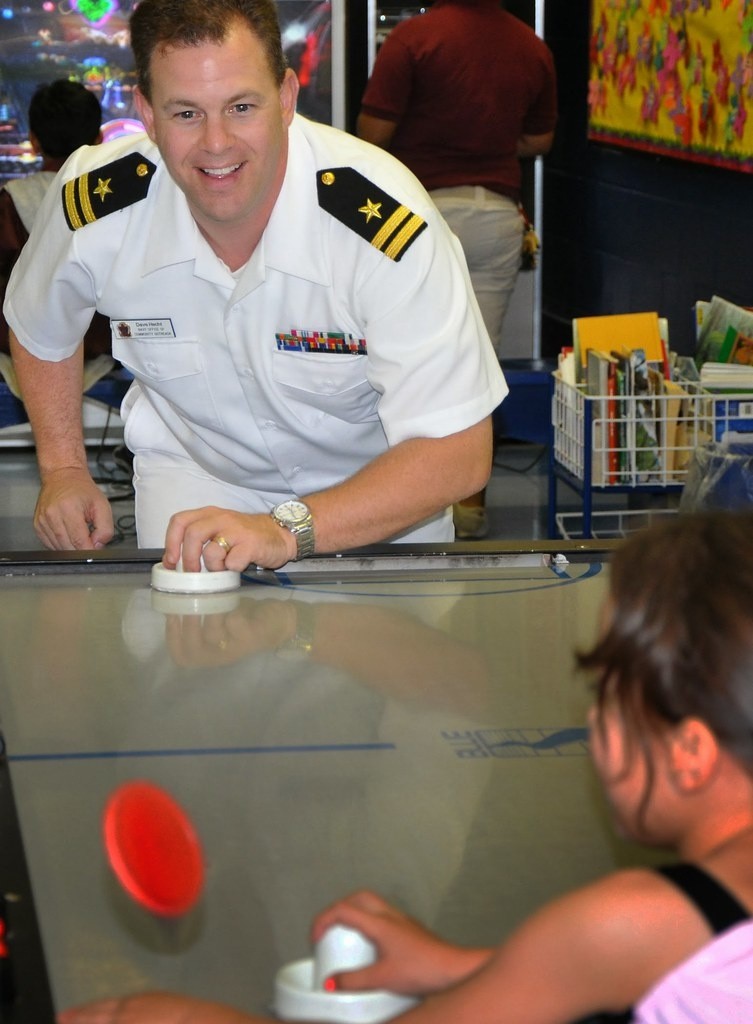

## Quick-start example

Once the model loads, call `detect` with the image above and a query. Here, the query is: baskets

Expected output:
[550,370,753,486]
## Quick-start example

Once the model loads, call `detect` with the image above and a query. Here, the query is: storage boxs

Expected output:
[493,358,556,441]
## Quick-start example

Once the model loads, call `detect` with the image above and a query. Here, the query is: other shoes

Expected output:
[453,502,489,539]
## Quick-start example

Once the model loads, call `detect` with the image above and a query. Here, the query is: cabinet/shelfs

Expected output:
[547,369,753,539]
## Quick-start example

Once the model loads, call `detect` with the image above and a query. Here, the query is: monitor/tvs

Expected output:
[0,0,345,183]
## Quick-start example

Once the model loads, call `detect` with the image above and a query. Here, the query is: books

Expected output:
[559,295,753,485]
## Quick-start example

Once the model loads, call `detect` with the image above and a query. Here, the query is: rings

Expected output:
[211,536,231,553]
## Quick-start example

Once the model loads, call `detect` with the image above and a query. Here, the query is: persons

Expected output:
[0,0,557,572]
[58,506,753,1024]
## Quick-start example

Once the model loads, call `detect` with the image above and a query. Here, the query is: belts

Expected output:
[486,182,521,202]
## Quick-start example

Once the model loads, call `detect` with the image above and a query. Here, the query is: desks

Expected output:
[0,539,753,1024]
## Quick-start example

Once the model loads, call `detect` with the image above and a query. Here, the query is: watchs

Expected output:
[270,498,316,562]
